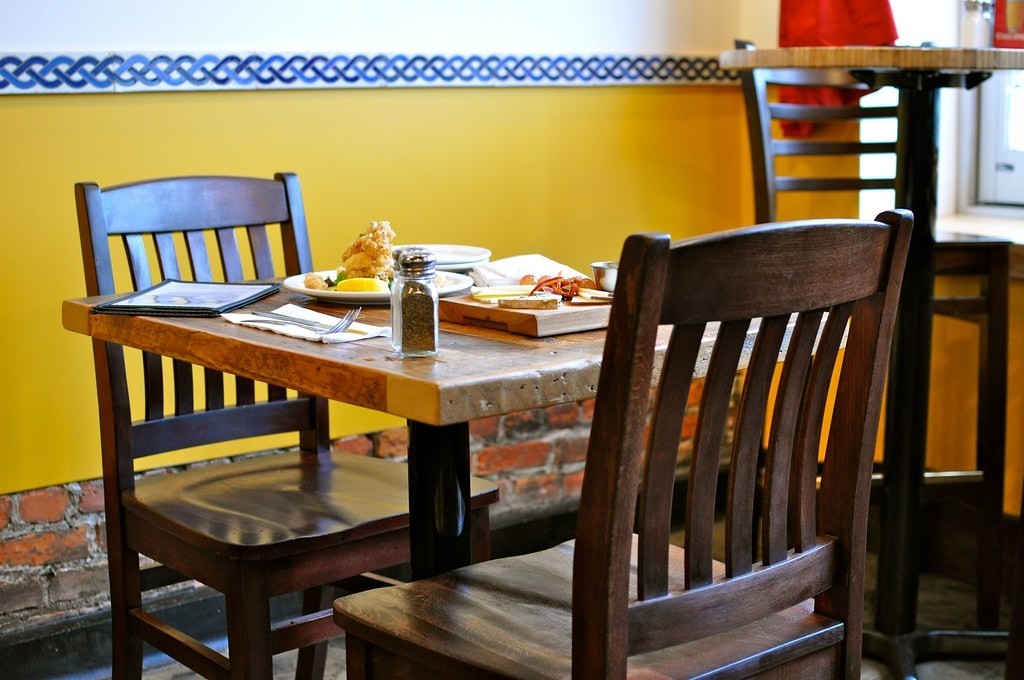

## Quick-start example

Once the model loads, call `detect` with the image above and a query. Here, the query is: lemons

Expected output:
[337,278,388,292]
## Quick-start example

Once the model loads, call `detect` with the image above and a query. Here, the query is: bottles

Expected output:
[960,0,995,48]
[390,247,440,358]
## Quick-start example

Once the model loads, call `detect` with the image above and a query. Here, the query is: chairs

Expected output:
[74,171,504,680]
[733,38,1016,665]
[329,207,919,680]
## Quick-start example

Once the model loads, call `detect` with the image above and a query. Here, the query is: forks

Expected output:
[240,306,363,335]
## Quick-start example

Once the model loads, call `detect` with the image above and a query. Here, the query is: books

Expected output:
[89,278,281,317]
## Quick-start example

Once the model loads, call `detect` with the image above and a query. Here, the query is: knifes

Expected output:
[251,311,368,336]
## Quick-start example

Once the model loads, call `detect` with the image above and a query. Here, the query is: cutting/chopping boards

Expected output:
[435,288,613,338]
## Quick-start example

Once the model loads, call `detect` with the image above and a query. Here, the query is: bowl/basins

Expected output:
[589,261,620,292]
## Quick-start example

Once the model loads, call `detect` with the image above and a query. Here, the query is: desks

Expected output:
[62,274,850,576]
[720,43,1024,680]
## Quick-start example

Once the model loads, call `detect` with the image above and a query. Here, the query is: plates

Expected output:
[392,245,492,272]
[282,270,475,305]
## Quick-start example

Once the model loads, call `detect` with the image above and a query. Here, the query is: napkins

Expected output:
[470,253,589,288]
[219,301,392,345]
